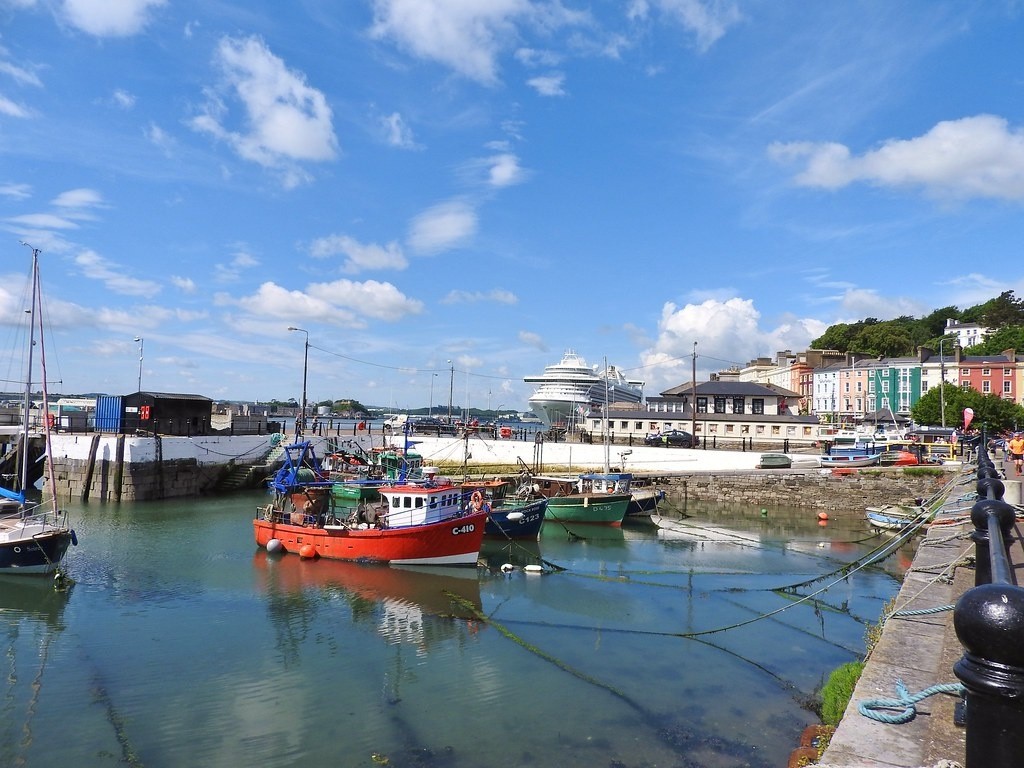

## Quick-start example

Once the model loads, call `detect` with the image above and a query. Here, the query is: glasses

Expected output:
[1015,436,1020,437]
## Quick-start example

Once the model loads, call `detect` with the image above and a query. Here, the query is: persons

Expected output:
[987,433,1024,475]
[294,413,318,436]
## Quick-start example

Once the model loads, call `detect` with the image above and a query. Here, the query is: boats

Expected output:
[253,356,666,569]
[819,441,945,468]
[863,506,932,531]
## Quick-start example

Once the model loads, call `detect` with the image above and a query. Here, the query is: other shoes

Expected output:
[1019,468,1022,472]
[1017,472,1019,476]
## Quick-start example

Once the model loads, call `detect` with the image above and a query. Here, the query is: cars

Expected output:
[643,430,700,449]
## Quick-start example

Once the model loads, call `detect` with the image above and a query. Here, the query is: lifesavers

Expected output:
[485,502,492,513]
[470,491,483,509]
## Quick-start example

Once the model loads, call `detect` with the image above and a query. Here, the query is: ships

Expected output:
[522,348,647,432]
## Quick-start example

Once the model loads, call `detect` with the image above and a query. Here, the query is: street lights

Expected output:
[447,359,454,424]
[287,326,308,435]
[428,373,439,418]
[23,309,33,432]
[939,337,956,428]
[851,356,856,442]
[691,341,698,449]
[134,337,143,393]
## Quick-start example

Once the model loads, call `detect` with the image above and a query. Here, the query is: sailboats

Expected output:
[0,239,77,578]
